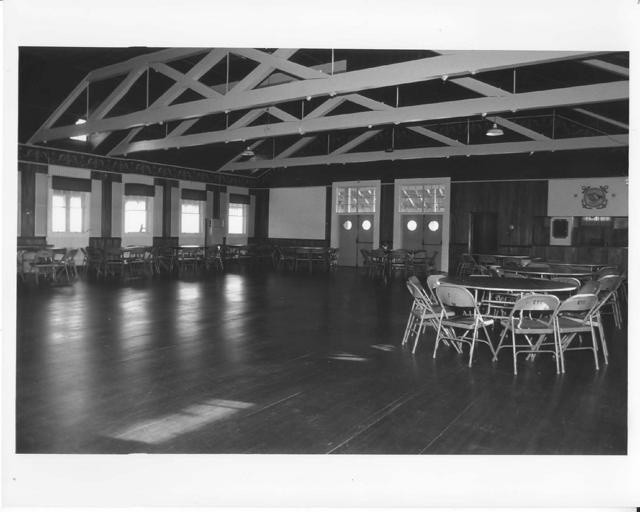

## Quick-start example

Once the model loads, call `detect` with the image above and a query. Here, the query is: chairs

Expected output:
[434,285,498,369]
[409,273,448,337]
[401,281,461,358]
[457,252,625,329]
[16,246,342,289]
[492,294,561,373]
[358,247,439,277]
[532,291,599,373]
[574,279,611,366]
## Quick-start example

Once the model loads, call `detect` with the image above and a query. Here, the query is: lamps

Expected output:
[240,146,255,156]
[485,117,504,137]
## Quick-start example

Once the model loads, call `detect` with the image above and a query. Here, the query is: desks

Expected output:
[465,277,577,345]
